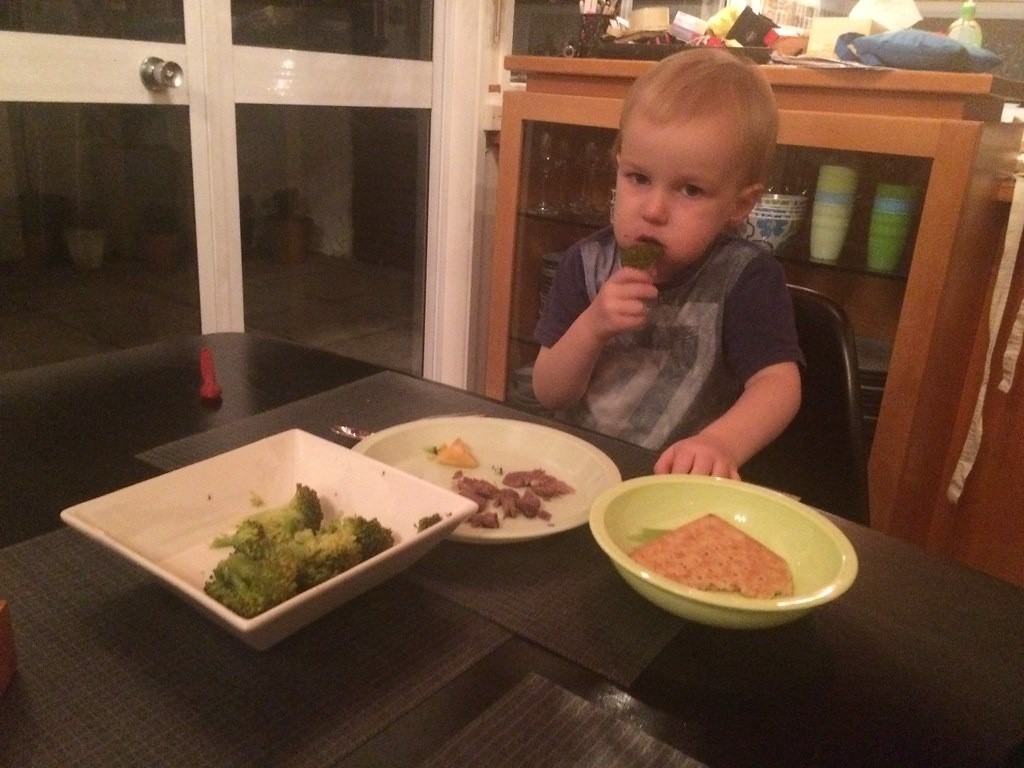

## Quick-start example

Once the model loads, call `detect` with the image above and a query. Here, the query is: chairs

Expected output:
[746,285,873,528]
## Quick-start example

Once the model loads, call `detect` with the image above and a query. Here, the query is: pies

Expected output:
[629,513,794,602]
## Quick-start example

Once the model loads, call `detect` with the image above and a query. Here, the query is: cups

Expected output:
[580,13,614,59]
[868,183,921,273]
[809,163,857,260]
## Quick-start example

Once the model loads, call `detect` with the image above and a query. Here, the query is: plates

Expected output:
[351,417,622,543]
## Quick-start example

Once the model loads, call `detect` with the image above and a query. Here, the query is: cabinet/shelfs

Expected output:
[488,54,1024,588]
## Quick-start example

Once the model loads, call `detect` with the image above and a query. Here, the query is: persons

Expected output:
[531,47,803,479]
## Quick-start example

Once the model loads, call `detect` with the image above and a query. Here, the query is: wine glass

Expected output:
[528,135,614,218]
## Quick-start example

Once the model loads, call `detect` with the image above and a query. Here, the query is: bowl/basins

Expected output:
[590,474,858,626]
[739,193,809,256]
[58,429,477,650]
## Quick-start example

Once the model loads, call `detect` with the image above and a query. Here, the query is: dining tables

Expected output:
[0,333,1023,768]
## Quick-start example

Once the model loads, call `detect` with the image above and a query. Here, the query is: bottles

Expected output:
[946,0,983,50]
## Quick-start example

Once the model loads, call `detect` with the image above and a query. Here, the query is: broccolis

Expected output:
[619,242,662,273]
[205,484,444,618]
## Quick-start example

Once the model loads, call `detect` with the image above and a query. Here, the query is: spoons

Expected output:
[328,424,370,441]
